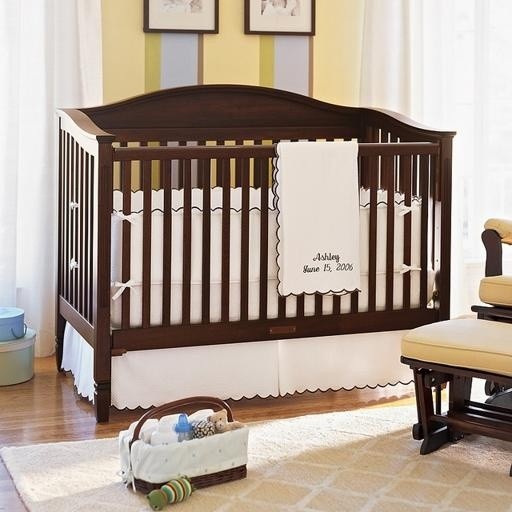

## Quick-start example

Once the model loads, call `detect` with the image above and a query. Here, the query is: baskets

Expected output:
[119,395,248,496]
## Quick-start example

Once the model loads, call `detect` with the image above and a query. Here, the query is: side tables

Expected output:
[400,318,512,477]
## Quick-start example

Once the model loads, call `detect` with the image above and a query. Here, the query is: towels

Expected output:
[271,141,362,298]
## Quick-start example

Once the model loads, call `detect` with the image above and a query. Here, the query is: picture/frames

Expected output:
[143,0,219,34]
[244,0,316,36]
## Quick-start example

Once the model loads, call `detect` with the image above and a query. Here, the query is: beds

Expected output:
[53,84,456,423]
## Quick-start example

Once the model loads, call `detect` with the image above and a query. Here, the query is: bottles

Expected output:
[176,415,192,442]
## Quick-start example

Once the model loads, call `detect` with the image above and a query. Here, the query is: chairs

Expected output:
[471,218,512,403]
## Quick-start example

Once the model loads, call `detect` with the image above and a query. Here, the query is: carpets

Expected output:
[0,403,512,512]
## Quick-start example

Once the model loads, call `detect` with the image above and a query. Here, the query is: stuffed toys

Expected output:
[145,475,196,510]
[206,409,243,433]
